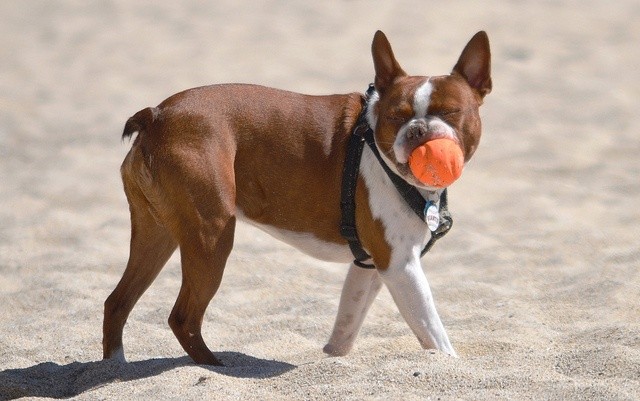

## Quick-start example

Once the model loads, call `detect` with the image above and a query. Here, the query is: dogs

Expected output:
[102,31,492,366]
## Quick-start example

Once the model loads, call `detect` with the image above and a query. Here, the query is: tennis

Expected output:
[409,138,465,187]
